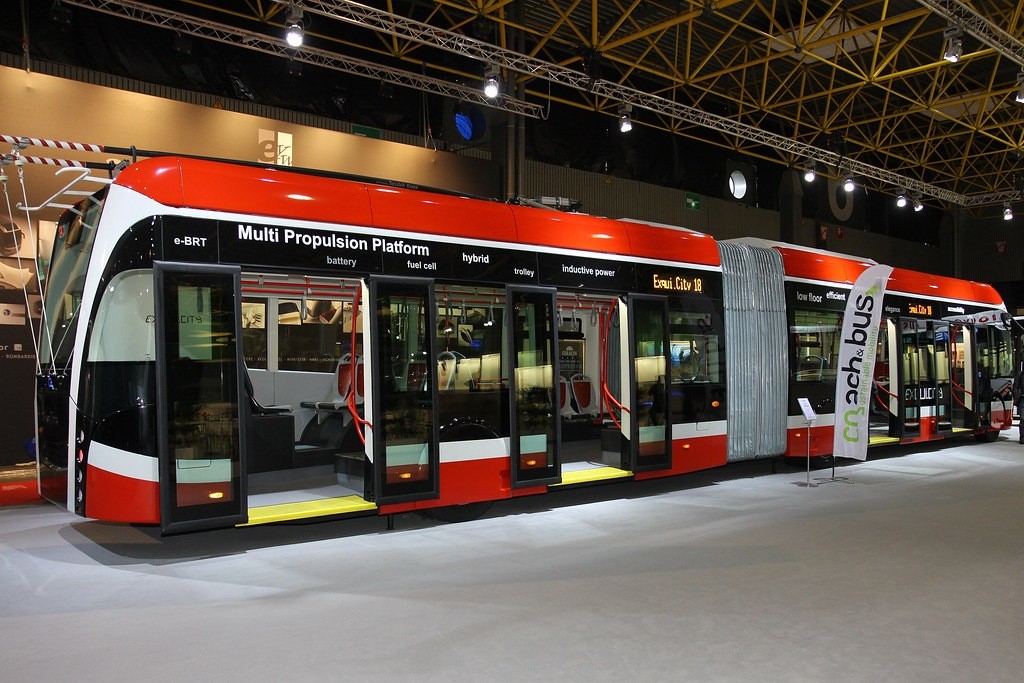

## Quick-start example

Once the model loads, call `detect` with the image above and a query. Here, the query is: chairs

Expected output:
[560,375,579,419]
[300,353,357,424]
[402,351,427,391]
[317,354,364,427]
[569,373,613,417]
[424,351,457,391]
[797,355,824,381]
[688,374,713,384]
[819,355,831,379]
[244,361,294,416]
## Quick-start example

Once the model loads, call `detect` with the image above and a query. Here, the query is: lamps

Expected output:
[913,199,923,212]
[844,179,855,192]
[896,195,906,208]
[286,25,305,48]
[1003,200,1013,220]
[804,169,815,183]
[619,117,633,133]
[484,78,498,98]
[1016,72,1024,103]
[942,18,963,62]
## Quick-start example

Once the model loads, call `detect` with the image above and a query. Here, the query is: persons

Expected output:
[648,377,665,425]
[1013,370,1024,444]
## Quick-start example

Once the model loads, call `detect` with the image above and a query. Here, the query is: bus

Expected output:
[0,133,1024,540]
